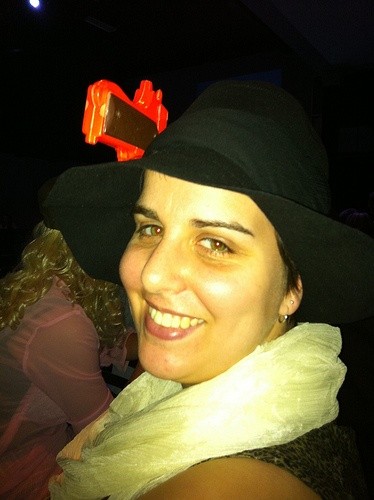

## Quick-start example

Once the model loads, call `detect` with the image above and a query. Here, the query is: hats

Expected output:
[36,78,373,325]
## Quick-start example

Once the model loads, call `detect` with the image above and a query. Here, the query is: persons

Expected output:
[38,76,374,499]
[1,218,144,499]
